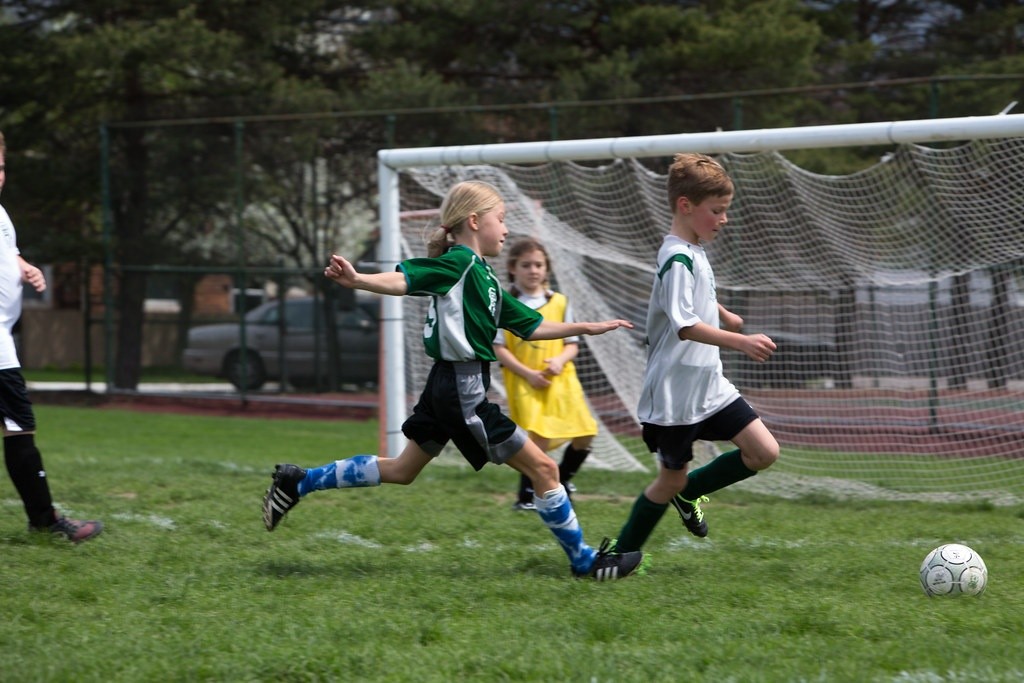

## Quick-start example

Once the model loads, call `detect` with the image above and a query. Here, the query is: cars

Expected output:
[182,287,379,396]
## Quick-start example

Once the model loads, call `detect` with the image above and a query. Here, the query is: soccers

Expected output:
[919,543,988,601]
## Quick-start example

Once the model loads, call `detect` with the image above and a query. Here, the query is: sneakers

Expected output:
[572,538,642,582]
[28,511,100,543]
[670,492,710,538]
[262,464,308,531]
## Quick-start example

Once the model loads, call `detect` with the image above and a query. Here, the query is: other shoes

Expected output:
[516,501,536,511]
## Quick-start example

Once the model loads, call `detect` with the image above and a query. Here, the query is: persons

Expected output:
[601,155,780,555]
[492,237,599,510]
[0,131,102,544]
[262,182,645,581]
